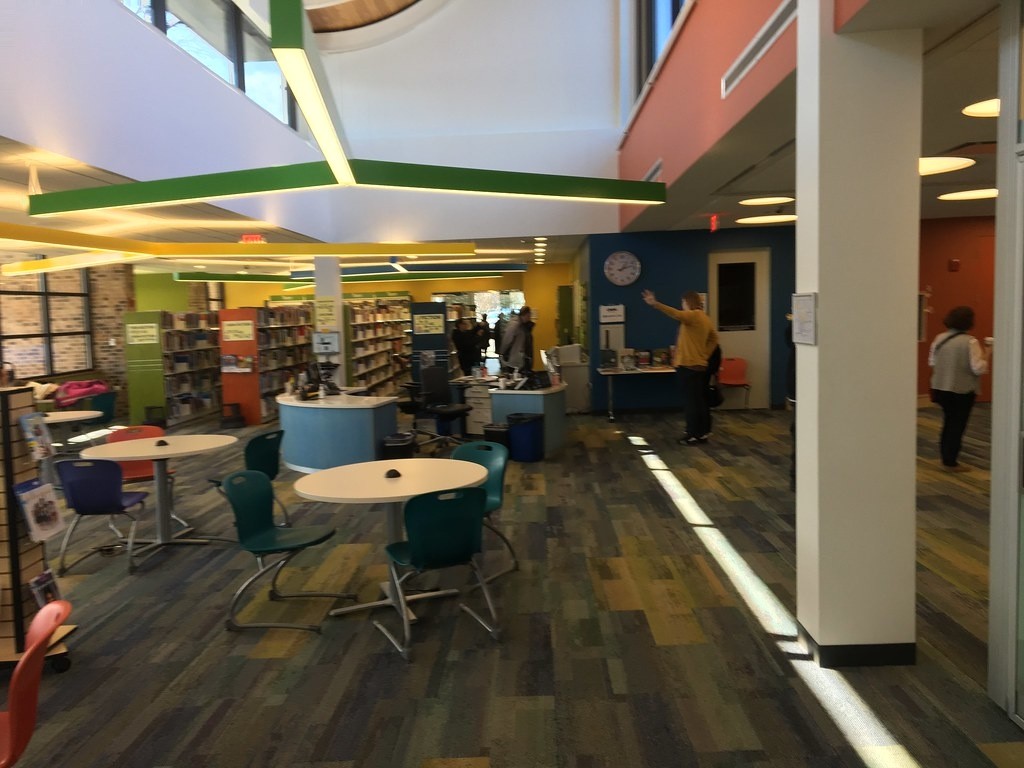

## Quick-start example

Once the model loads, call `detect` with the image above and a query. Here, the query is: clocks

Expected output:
[604,250,641,285]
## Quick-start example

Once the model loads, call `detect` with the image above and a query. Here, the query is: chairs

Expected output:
[0,382,518,768]
[714,357,752,413]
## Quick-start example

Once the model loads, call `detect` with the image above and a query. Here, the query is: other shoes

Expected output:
[945,463,971,473]
[680,432,708,445]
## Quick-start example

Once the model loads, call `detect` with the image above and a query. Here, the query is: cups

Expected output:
[498,378,507,389]
[983,337,992,347]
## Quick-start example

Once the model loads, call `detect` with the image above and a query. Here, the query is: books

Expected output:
[162,312,221,351]
[353,336,412,388]
[18,412,57,462]
[164,349,222,418]
[446,305,477,319]
[14,477,66,540]
[255,305,314,348]
[616,345,677,367]
[350,305,412,340]
[258,346,314,417]
[29,569,65,608]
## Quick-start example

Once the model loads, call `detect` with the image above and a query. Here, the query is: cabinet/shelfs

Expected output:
[448,376,568,460]
[124,296,476,427]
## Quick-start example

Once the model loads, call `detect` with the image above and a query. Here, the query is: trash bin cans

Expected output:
[385,432,413,459]
[507,412,544,462]
[435,384,466,435]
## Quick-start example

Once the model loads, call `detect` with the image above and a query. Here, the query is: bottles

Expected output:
[318,384,326,398]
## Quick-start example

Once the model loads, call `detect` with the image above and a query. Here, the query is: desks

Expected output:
[42,411,103,453]
[596,365,678,422]
[275,387,399,474]
[293,457,507,664]
[79,434,239,574]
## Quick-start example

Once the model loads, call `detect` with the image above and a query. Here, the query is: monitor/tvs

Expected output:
[484,357,501,375]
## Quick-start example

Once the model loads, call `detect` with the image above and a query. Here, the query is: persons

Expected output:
[479,314,490,355]
[926,306,990,472]
[446,321,464,379]
[641,289,719,447]
[499,305,536,372]
[452,318,485,376]
[784,314,796,492]
[494,310,518,369]
